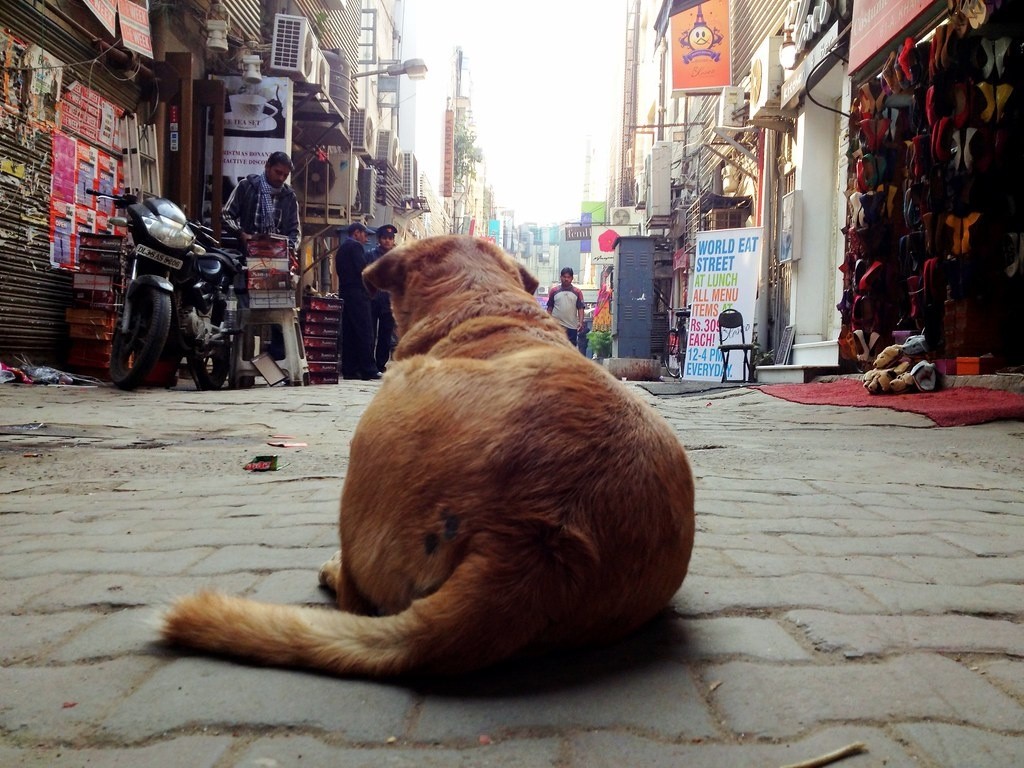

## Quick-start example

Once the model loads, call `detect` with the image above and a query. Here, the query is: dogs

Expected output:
[145,234,695,677]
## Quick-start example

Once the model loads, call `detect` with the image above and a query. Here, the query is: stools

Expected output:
[228,308,310,390]
[649,311,670,367]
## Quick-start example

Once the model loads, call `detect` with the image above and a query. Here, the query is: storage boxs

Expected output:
[301,295,344,384]
[956,356,1003,375]
[64,232,134,369]
[246,239,297,308]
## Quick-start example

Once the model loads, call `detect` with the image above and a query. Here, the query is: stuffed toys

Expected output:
[861,327,936,394]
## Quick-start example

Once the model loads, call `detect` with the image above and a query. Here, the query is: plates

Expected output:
[221,113,277,131]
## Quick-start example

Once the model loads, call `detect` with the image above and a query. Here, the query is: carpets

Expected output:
[635,379,770,401]
[748,377,1024,426]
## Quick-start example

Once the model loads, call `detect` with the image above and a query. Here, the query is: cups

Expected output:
[229,95,279,127]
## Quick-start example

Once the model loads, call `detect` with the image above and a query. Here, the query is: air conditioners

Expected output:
[609,205,645,236]
[403,151,420,209]
[269,12,318,83]
[376,130,403,179]
[749,36,785,117]
[712,86,744,142]
[291,155,359,203]
[293,48,331,111]
[349,109,378,157]
[645,140,672,222]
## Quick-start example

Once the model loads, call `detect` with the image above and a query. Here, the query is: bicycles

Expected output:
[661,310,692,378]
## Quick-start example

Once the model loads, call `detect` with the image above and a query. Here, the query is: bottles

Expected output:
[227,285,237,327]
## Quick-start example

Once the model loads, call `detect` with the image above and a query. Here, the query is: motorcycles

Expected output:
[83,185,248,391]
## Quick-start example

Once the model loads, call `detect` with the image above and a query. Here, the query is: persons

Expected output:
[363,223,397,372]
[335,223,386,379]
[576,319,590,356]
[546,267,585,347]
[220,151,303,389]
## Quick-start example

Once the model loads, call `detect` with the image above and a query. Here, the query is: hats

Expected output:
[376,224,397,236]
[349,222,376,236]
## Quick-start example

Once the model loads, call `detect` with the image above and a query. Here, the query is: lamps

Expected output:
[241,54,265,84]
[203,4,231,53]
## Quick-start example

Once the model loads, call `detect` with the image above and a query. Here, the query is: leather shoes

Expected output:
[371,372,385,379]
[343,375,361,380]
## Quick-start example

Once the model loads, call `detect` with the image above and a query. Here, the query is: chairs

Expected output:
[717,308,758,383]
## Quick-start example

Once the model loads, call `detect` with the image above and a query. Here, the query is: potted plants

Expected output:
[585,330,614,365]
